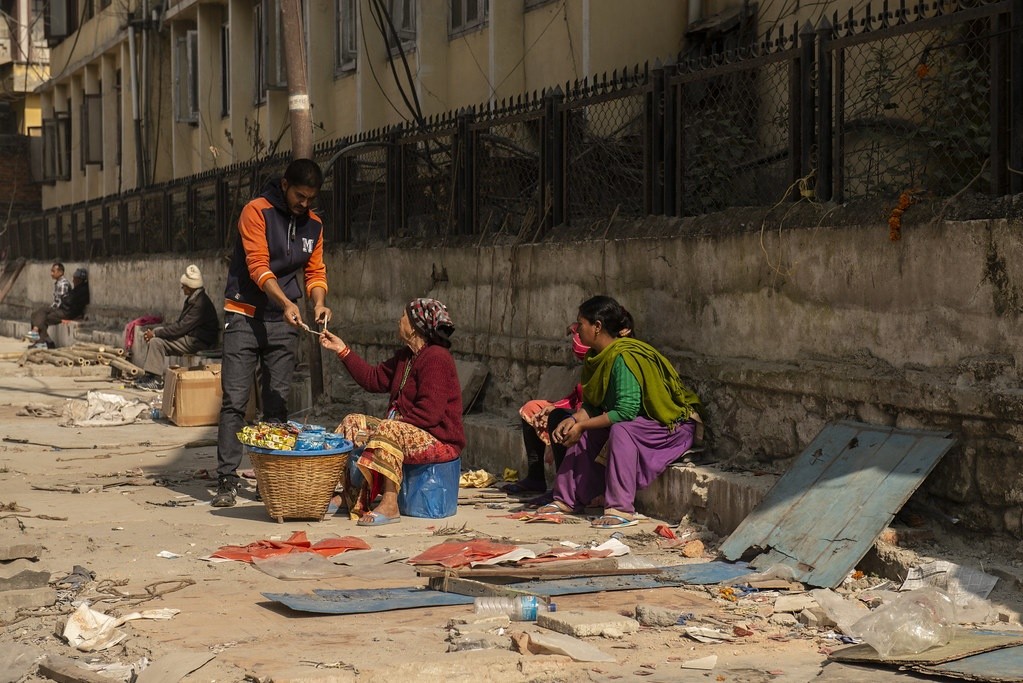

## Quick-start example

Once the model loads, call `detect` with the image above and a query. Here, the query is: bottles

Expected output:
[473,595,557,621]
[136,408,166,419]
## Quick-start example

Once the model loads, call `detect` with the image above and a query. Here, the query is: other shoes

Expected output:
[24,331,40,339]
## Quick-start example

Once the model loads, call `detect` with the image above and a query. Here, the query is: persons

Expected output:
[211,158,333,507]
[131,264,219,391]
[501,321,592,509]
[535,295,704,528]
[24,262,90,350]
[318,298,467,526]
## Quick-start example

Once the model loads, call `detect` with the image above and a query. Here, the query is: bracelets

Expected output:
[336,343,350,358]
[562,415,577,424]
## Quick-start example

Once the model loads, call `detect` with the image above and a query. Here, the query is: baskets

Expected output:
[245,439,353,523]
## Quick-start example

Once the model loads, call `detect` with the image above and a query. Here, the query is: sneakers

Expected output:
[136,378,164,392]
[255,484,263,500]
[211,476,238,506]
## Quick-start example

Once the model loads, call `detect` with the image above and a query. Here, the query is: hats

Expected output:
[73,269,86,280]
[181,264,203,288]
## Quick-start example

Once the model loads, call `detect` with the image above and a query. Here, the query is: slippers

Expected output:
[529,496,552,505]
[357,511,401,526]
[500,484,547,494]
[536,504,573,515]
[326,501,348,514]
[592,516,639,528]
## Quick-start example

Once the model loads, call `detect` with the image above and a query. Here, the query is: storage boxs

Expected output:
[161,364,263,427]
